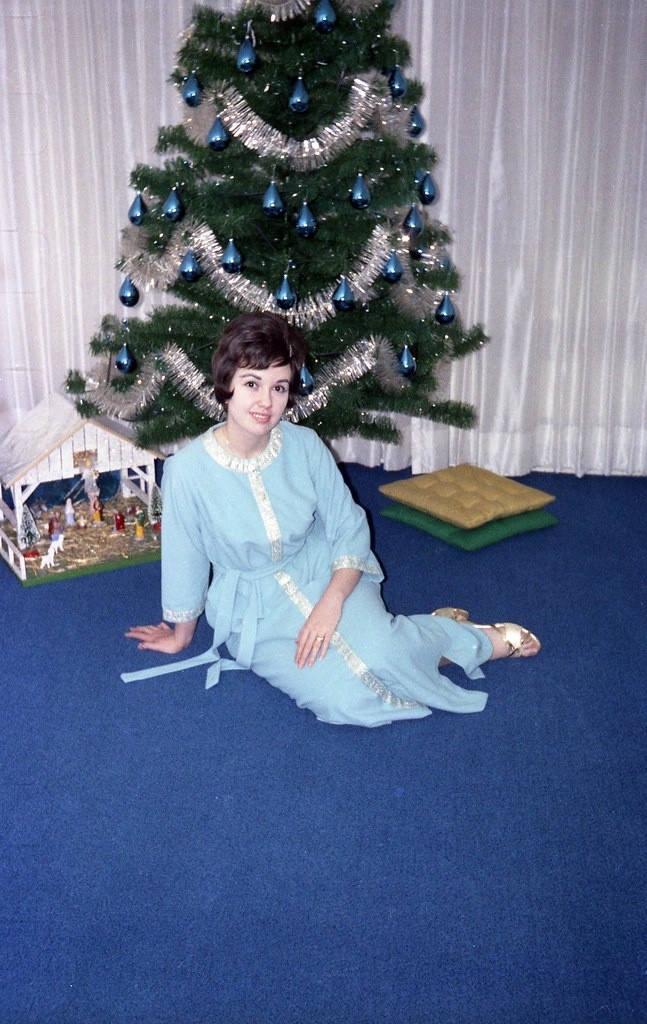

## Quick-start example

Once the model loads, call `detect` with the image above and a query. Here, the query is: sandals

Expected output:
[461,621,542,658]
[430,608,468,623]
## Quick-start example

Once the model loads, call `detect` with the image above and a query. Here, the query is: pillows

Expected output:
[379,463,557,529]
[379,503,557,551]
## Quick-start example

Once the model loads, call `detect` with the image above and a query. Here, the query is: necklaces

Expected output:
[221,425,236,458]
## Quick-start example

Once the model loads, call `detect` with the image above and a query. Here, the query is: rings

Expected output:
[316,636,324,642]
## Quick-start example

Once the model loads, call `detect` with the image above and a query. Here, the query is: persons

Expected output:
[133,505,146,542]
[93,496,105,522]
[65,498,77,527]
[151,512,162,532]
[120,310,540,728]
[48,510,65,541]
[114,510,125,531]
